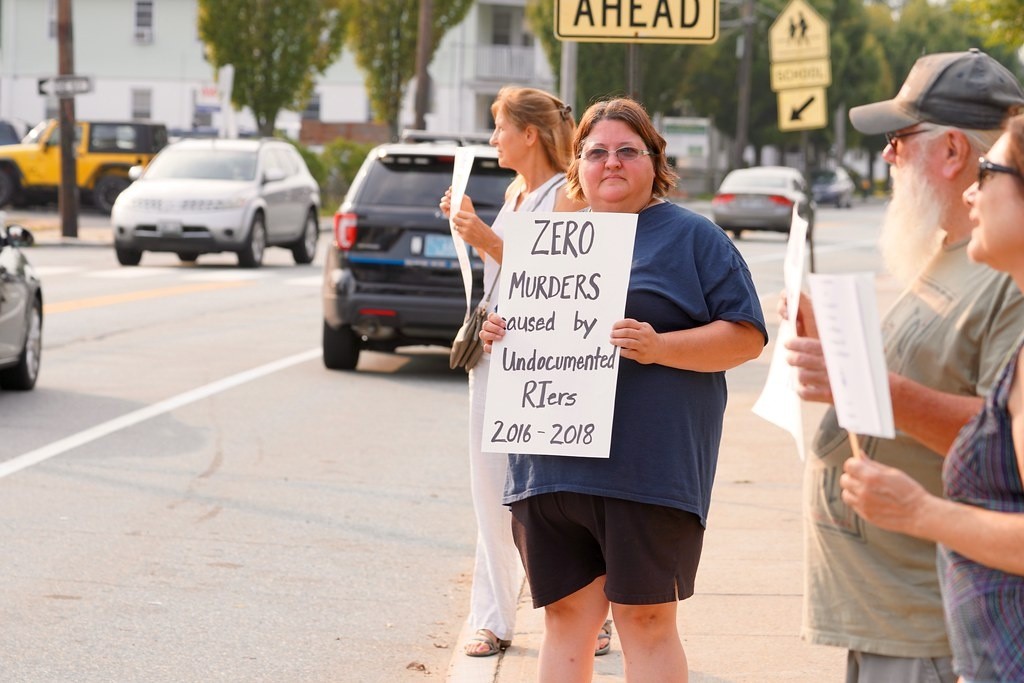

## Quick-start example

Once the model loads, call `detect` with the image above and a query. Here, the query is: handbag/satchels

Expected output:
[449,307,488,370]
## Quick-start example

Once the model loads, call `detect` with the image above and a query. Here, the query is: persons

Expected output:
[840,115,1024,683]
[776,47,1024,683]
[439,86,613,657]
[479,98,770,683]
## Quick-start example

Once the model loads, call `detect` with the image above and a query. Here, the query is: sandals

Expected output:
[465,628,513,656]
[593,620,611,655]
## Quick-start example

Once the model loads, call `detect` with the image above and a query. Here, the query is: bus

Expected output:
[652,112,722,175]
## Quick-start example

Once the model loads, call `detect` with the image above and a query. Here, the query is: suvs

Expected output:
[0,117,170,217]
[319,130,519,371]
[107,136,321,270]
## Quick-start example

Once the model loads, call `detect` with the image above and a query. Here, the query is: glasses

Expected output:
[580,141,655,161]
[976,157,1024,190]
[884,128,935,151]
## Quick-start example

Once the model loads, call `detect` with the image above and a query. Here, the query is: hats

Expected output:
[849,47,1024,135]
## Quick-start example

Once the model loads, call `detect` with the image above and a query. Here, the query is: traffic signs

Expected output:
[37,75,94,96]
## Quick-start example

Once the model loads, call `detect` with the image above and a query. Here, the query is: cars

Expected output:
[0,224,45,390]
[812,168,857,209]
[711,166,816,239]
